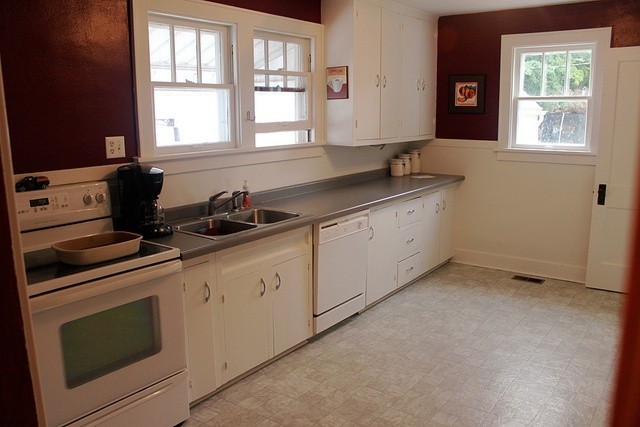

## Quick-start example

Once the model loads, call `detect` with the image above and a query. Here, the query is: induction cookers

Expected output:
[14,230,183,298]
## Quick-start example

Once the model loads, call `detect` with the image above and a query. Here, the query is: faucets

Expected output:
[229,190,243,212]
[196,188,249,220]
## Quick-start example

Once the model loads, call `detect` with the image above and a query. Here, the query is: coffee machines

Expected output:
[117,163,173,238]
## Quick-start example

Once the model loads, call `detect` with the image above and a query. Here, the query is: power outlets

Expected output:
[104,137,125,159]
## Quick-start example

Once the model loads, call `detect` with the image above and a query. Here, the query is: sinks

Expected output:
[223,209,300,226]
[173,217,258,238]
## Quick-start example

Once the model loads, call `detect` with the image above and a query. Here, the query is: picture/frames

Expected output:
[447,74,486,116]
[326,66,348,99]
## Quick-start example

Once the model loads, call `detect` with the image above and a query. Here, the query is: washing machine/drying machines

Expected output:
[14,178,194,427]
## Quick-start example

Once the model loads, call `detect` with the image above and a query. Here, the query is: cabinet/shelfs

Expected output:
[353,2,406,141]
[222,229,313,387]
[407,15,435,137]
[368,207,397,305]
[426,189,454,271]
[182,262,215,404]
[398,198,427,287]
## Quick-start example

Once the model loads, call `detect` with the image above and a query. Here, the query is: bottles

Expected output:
[398,153,411,175]
[390,158,405,176]
[409,150,421,173]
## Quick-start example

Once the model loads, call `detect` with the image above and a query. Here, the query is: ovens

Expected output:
[29,260,188,427]
